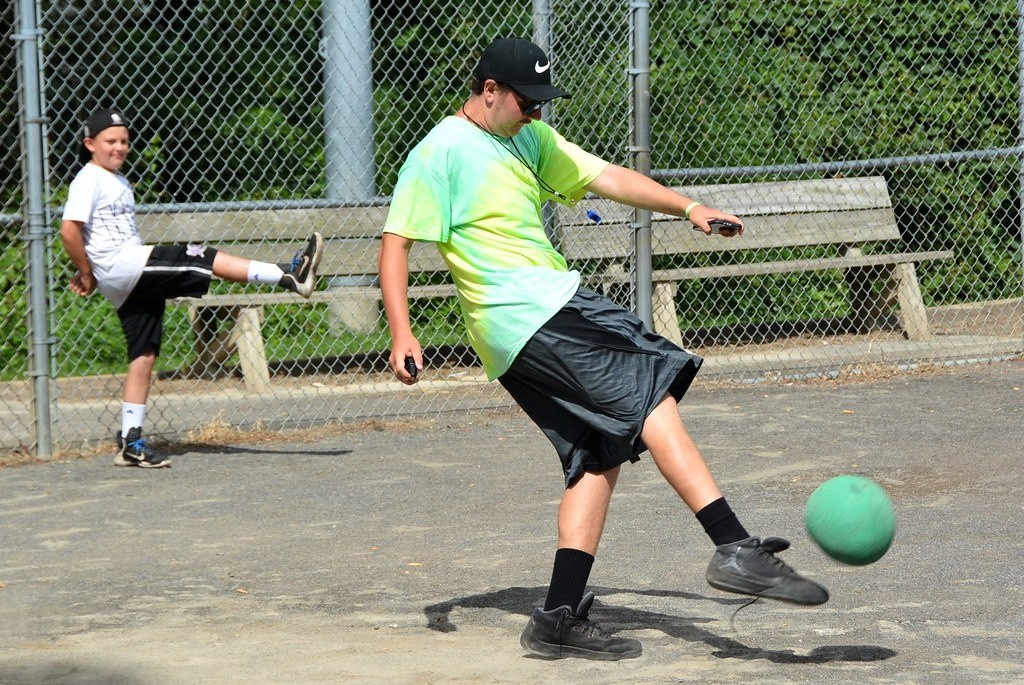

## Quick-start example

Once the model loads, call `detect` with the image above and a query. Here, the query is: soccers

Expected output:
[806,475,896,566]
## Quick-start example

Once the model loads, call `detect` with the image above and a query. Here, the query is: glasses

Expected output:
[508,84,550,116]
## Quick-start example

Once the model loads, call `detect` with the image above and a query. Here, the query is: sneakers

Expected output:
[277,231,323,298]
[520,590,643,660]
[707,535,830,606]
[113,427,172,468]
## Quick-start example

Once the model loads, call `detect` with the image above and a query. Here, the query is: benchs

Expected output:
[134,206,458,391]
[554,175,954,350]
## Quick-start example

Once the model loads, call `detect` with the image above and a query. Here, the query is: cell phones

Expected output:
[691,220,741,234]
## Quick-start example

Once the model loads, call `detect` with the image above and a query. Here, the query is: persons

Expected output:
[377,37,830,660]
[60,110,323,468]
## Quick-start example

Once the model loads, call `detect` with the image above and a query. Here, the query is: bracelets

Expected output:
[684,202,702,220]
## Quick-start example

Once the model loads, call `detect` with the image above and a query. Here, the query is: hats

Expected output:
[477,36,573,102]
[79,110,127,162]
